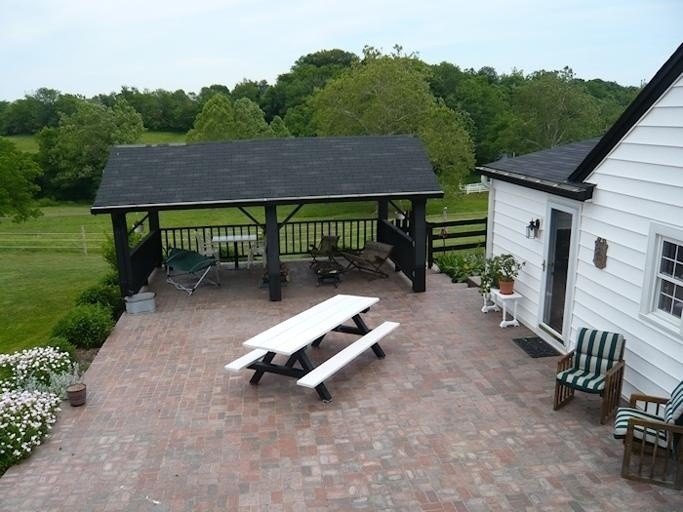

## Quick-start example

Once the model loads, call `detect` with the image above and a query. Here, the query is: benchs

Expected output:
[226,321,401,404]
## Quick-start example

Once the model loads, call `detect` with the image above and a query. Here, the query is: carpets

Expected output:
[512,336,563,359]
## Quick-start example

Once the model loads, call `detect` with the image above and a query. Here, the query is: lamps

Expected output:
[525,219,541,240]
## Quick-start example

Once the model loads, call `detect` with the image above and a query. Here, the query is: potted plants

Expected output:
[131,212,144,233]
[393,199,406,220]
[499,259,528,294]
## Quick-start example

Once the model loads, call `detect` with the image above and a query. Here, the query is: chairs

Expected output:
[553,326,682,492]
[164,246,222,297]
[247,240,267,271]
[309,235,395,277]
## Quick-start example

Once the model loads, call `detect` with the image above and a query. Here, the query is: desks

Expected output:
[211,235,257,272]
[481,287,522,329]
[241,292,380,404]
[313,264,344,289]
